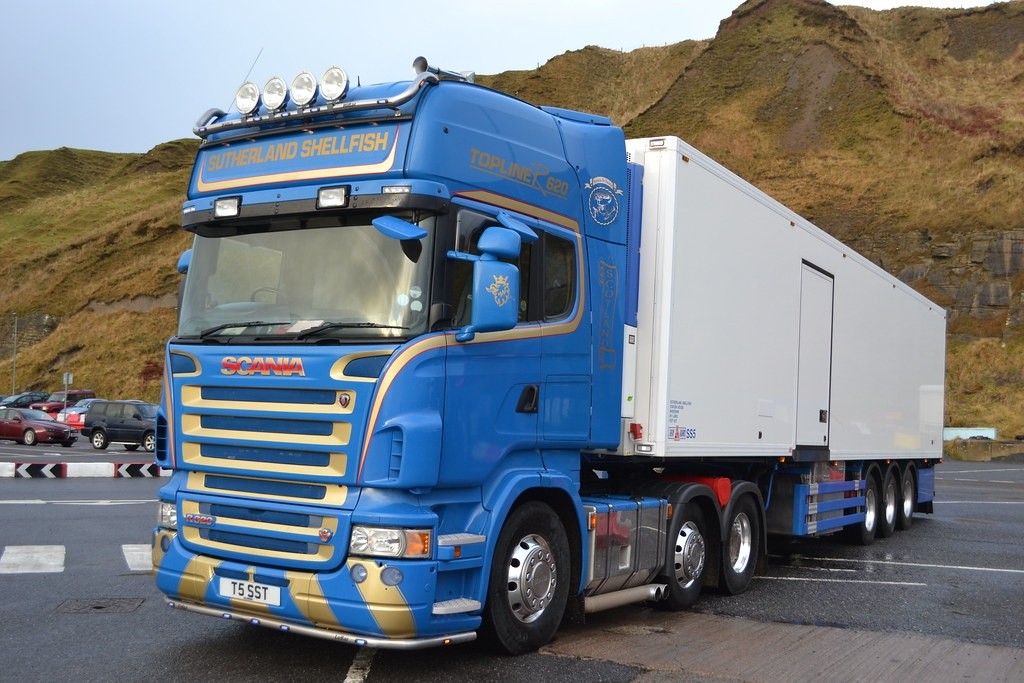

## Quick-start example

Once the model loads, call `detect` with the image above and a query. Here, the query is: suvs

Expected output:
[81,399,160,453]
[30,389,95,416]
[0,391,50,411]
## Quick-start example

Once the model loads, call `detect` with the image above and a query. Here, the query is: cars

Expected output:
[1,408,78,447]
[56,398,108,432]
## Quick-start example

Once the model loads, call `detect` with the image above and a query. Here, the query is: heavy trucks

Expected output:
[150,55,947,656]
